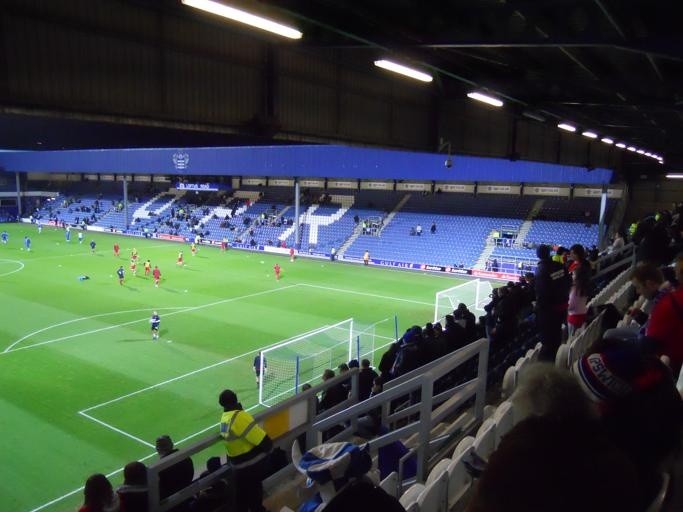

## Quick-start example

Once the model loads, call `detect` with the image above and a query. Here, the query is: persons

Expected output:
[485,200,683,355]
[253,351,267,384]
[218,390,273,466]
[79,435,222,511]
[294,302,682,511]
[353,186,443,266]
[1,184,332,288]
[150,311,160,339]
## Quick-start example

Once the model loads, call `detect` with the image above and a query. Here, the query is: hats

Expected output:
[301,442,372,483]
[403,328,416,343]
[569,334,660,405]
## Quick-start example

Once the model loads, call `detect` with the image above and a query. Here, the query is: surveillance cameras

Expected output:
[445,160,452,169]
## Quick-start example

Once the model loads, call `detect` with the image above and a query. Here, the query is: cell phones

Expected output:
[623,308,631,315]
